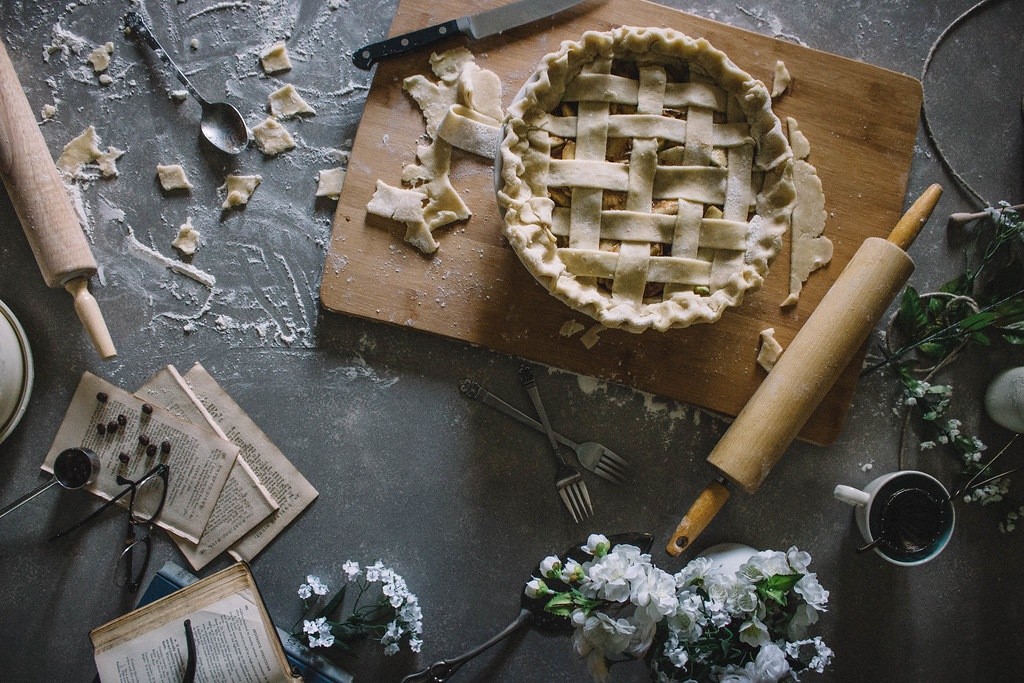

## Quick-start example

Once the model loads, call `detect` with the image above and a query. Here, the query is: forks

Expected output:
[517,365,594,524]
[458,377,633,484]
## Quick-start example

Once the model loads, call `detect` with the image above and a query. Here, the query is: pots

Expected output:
[0,300,36,448]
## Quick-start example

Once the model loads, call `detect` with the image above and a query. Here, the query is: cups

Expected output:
[833,470,956,568]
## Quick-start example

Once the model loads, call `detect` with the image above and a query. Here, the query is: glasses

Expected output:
[46,462,169,593]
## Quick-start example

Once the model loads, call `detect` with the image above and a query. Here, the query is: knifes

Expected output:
[352,0,585,72]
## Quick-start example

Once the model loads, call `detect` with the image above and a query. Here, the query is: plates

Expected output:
[493,70,595,319]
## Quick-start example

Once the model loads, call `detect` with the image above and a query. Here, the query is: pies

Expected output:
[495,26,799,337]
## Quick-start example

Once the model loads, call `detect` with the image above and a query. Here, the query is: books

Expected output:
[92,563,354,683]
[89,562,303,683]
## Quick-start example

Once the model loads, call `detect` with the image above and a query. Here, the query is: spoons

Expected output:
[123,11,250,154]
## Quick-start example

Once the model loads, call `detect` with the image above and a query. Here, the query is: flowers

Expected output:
[856,200,1023,532]
[283,558,426,660]
[519,531,835,682]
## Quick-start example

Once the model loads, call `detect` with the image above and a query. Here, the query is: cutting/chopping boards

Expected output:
[318,0,923,448]
